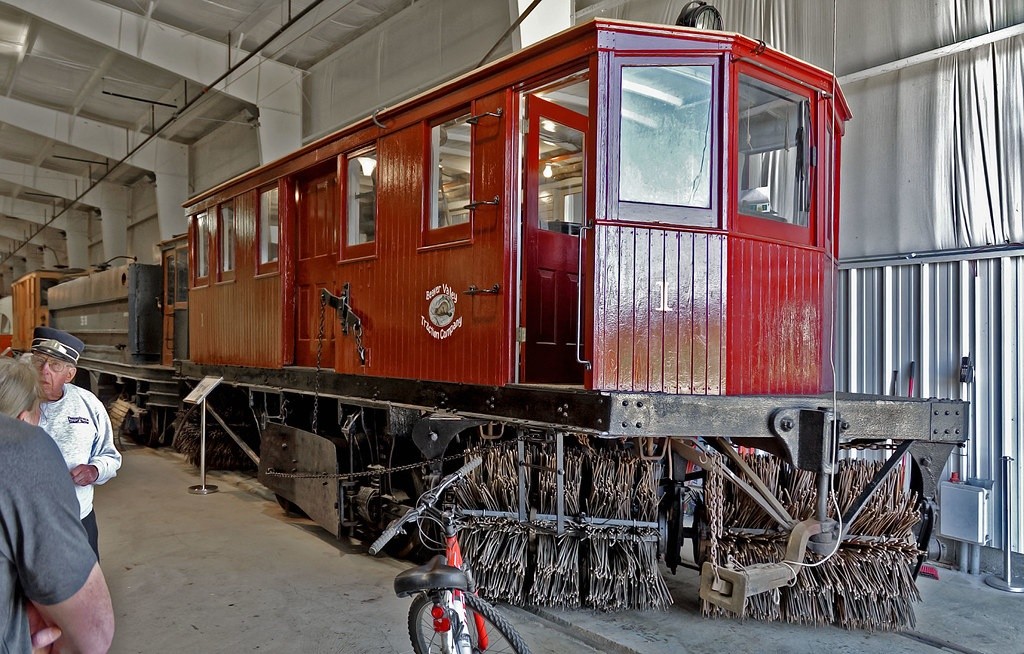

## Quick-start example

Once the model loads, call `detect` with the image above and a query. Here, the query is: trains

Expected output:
[11,17,972,631]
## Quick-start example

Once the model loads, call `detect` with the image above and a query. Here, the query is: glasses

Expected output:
[33,354,71,372]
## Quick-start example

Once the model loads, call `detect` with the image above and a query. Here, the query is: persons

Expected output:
[0,356,42,426]
[30,327,122,564]
[0,412,115,654]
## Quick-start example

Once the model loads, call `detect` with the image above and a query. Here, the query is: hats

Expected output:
[31,327,85,367]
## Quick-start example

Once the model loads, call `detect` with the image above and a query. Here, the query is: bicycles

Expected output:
[368,457,535,654]
